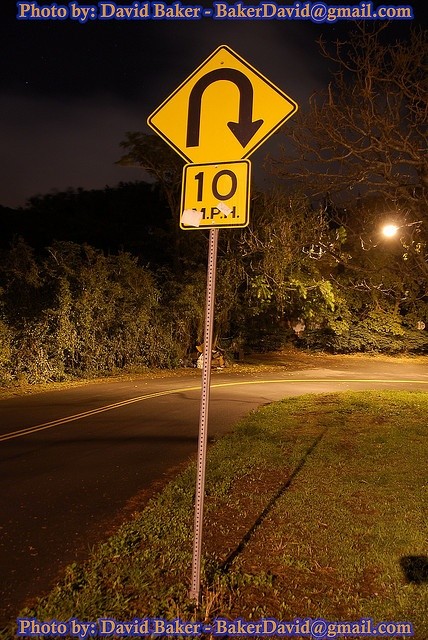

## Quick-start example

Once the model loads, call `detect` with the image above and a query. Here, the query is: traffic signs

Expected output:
[180,160,250,230]
